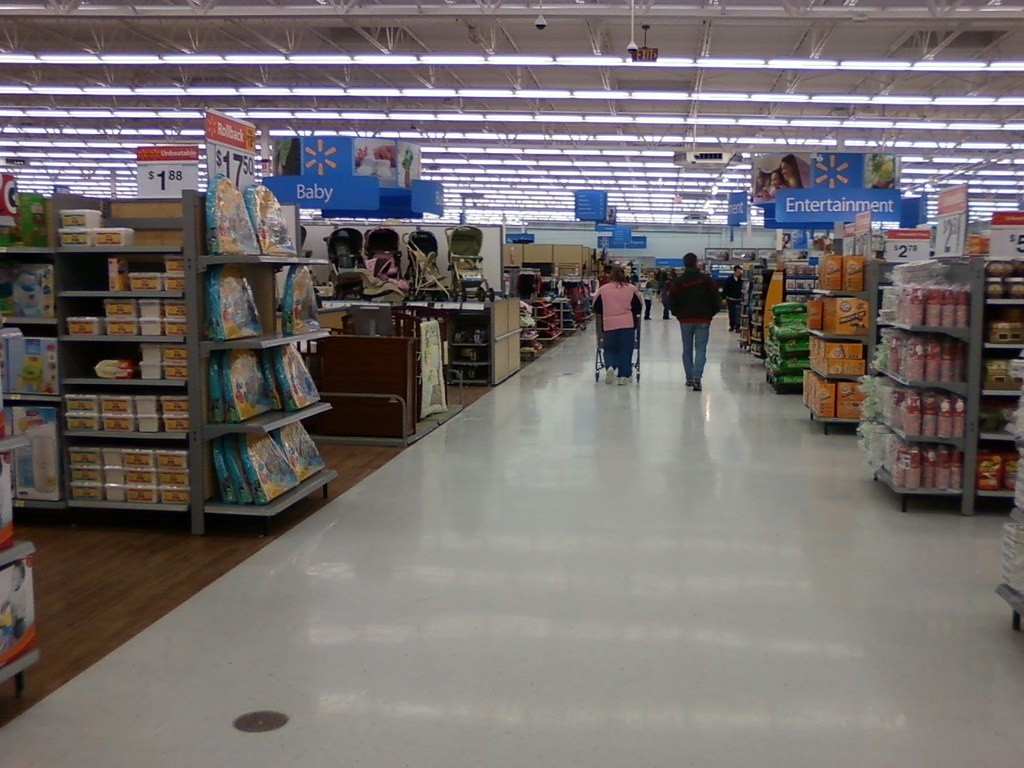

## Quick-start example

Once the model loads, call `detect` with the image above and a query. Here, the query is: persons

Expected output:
[644,282,652,320]
[669,253,720,391]
[654,268,677,295]
[660,279,672,320]
[754,154,803,201]
[721,265,743,331]
[592,266,642,385]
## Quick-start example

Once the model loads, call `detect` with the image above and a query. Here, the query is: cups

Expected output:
[889,334,965,382]
[890,388,965,438]
[897,287,969,326]
[895,447,962,489]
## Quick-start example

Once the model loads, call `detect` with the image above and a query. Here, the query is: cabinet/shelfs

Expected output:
[695,251,821,365]
[2,324,41,702]
[869,252,1024,516]
[607,258,687,298]
[408,297,521,385]
[801,260,909,435]
[1,188,336,532]
[503,238,610,362]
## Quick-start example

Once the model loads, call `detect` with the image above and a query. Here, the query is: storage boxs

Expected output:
[785,252,870,418]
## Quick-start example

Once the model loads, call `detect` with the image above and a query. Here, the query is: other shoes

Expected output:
[685,380,693,386]
[729,328,734,332]
[605,367,615,384]
[617,377,629,385]
[644,318,652,320]
[736,329,741,333]
[663,316,670,319]
[693,380,701,391]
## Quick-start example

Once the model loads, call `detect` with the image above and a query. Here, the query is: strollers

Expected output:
[364,227,410,298]
[324,228,368,299]
[402,231,450,300]
[442,226,494,303]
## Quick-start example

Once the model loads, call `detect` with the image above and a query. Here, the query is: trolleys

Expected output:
[595,315,641,383]
